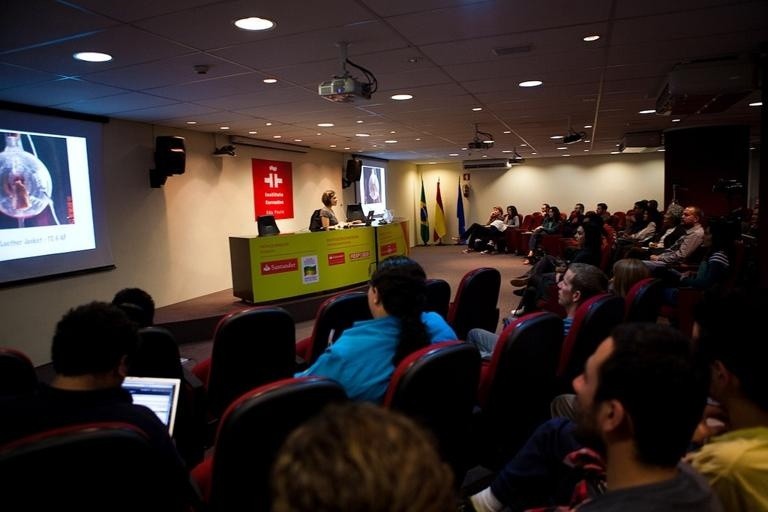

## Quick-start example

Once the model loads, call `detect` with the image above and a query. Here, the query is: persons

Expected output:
[610,200,736,302]
[320,190,338,231]
[678,284,766,508]
[273,402,452,511]
[571,322,725,510]
[451,206,520,255]
[465,263,608,362]
[735,207,761,246]
[293,255,457,404]
[455,393,604,510]
[510,203,609,317]
[3,288,186,510]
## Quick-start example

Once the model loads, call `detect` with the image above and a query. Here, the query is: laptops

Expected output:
[350,211,374,227]
[122,377,182,438]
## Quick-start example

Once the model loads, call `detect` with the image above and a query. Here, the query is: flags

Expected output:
[434,184,447,242]
[456,184,467,245]
[420,184,429,242]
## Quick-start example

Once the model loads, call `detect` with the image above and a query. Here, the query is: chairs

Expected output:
[477,210,720,273]
[423,279,450,322]
[295,291,372,368]
[195,305,294,418]
[565,297,625,366]
[381,342,481,457]
[449,266,501,334]
[346,204,365,220]
[628,278,665,326]
[190,377,348,512]
[483,311,565,415]
[3,422,152,512]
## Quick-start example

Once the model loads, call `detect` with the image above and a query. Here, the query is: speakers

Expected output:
[156,135,186,174]
[346,160,361,181]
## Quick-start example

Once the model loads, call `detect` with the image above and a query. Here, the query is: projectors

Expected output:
[318,78,371,104]
[563,133,582,145]
[509,157,525,163]
[468,140,495,149]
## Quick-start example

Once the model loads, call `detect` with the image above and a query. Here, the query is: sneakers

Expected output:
[511,253,535,316]
[452,237,495,254]
